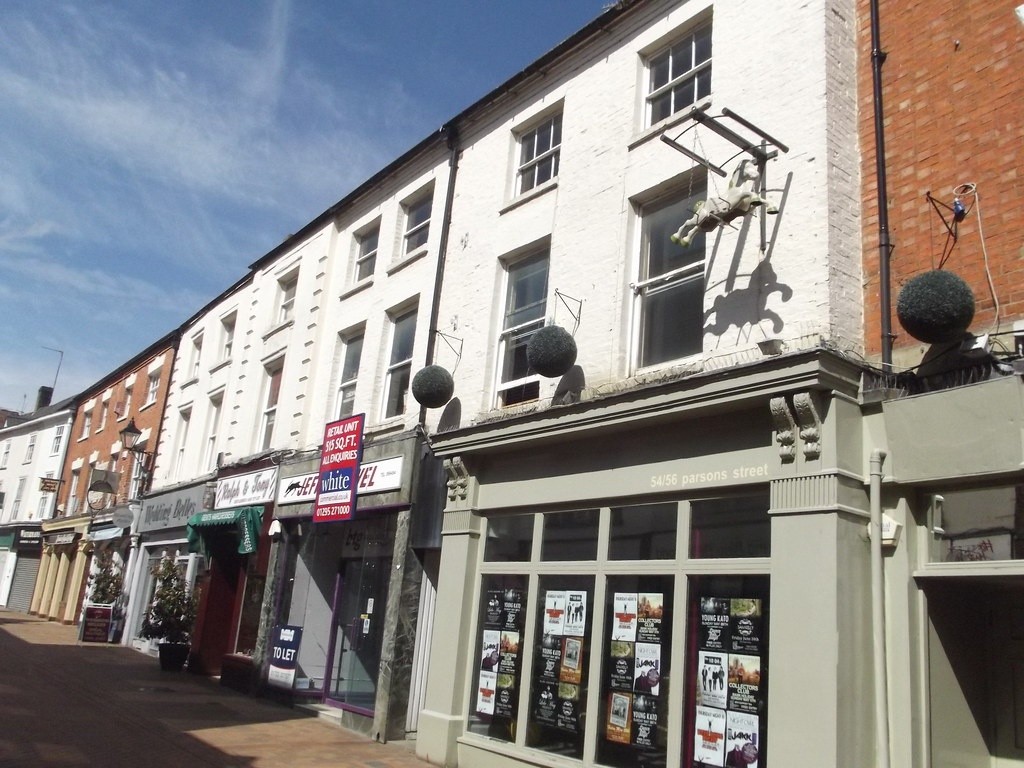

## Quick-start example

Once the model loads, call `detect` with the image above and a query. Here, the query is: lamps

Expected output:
[119,416,151,474]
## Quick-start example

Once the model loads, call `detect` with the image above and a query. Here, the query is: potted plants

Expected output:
[137,554,203,674]
[79,547,124,607]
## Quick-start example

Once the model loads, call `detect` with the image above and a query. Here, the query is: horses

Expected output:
[670,158,780,251]
